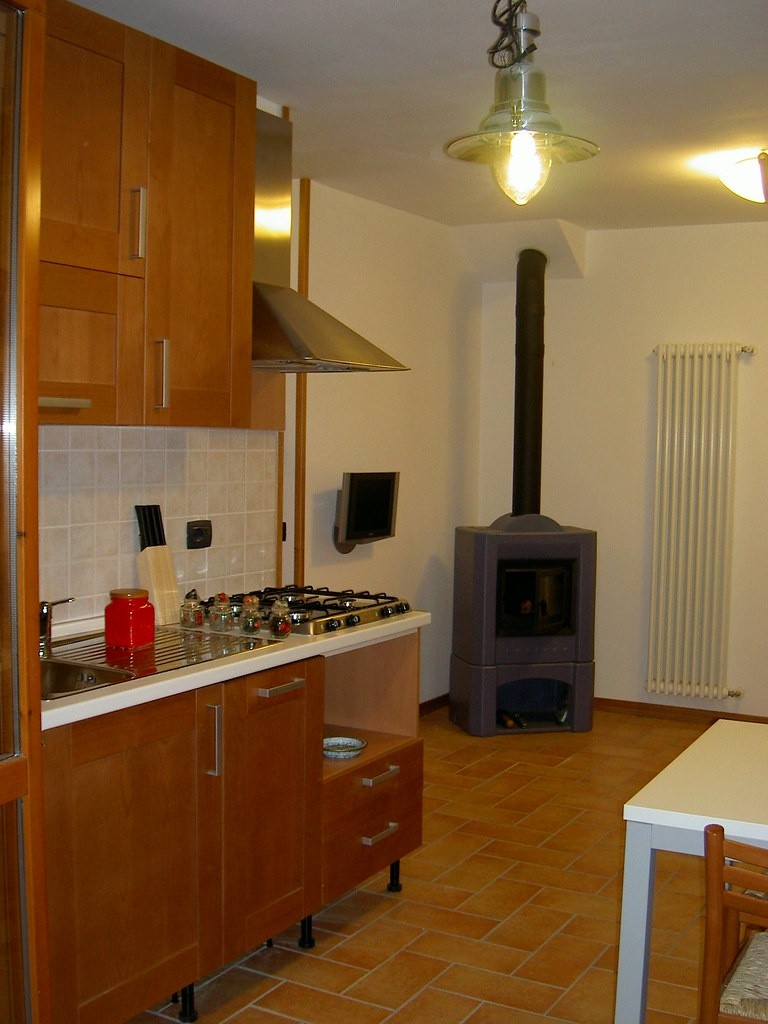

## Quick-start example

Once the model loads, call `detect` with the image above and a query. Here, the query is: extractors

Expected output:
[252,108,412,373]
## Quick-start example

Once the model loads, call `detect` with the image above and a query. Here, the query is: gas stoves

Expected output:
[200,585,411,635]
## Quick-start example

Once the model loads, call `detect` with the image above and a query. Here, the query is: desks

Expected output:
[612,721,768,1024]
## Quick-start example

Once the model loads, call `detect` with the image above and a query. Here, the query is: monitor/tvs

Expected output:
[335,473,400,543]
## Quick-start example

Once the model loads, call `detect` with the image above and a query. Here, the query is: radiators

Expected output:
[644,341,755,700]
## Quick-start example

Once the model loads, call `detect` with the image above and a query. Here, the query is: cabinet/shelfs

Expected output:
[41,626,424,1021]
[0,1,259,433]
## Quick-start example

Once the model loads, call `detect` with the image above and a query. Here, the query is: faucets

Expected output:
[40,598,75,658]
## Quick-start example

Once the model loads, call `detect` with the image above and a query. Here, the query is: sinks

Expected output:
[40,659,131,693]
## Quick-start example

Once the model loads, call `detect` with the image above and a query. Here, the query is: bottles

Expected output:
[209,593,235,631]
[104,588,155,648]
[180,589,205,627]
[239,597,262,634]
[269,601,292,639]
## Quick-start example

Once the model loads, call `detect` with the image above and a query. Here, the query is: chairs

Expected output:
[700,824,768,1024]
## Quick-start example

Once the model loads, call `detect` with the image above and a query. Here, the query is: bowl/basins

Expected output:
[322,736,367,759]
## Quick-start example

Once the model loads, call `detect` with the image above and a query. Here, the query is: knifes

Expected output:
[135,505,166,552]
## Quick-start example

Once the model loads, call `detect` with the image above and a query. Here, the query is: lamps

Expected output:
[717,151,768,205]
[446,0,601,206]
